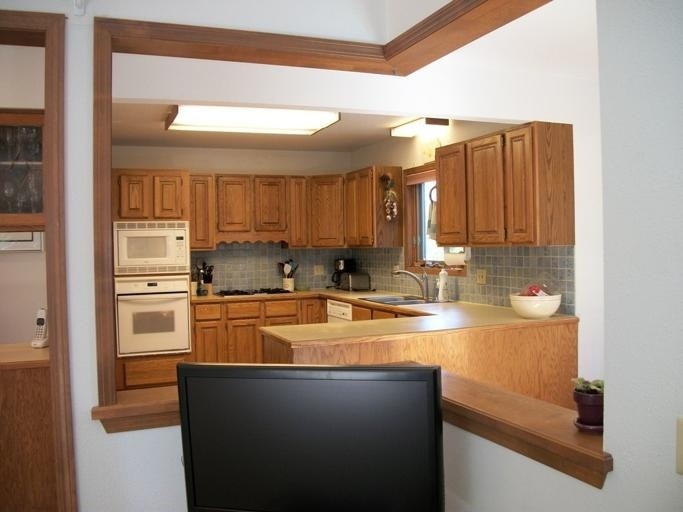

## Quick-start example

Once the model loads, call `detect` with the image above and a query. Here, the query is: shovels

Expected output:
[277,262,291,278]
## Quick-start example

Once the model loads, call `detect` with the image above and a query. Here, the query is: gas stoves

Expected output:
[215,287,291,298]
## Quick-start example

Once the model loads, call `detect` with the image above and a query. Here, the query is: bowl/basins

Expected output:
[506,291,562,321]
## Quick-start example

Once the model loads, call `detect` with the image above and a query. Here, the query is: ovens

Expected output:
[112,220,191,358]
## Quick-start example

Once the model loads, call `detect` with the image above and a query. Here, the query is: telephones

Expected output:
[31,307,49,349]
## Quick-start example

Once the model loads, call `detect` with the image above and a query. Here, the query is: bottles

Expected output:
[437,268,450,302]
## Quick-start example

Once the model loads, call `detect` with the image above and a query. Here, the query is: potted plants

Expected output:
[571,372,603,435]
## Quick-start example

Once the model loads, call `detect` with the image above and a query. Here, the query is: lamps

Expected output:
[159,105,342,137]
[388,116,450,140]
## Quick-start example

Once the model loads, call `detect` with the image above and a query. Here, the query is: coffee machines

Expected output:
[330,258,354,290]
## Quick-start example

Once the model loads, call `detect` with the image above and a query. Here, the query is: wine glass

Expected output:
[0,126,41,215]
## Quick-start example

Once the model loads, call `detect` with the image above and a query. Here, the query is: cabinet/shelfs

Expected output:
[110,165,191,223]
[190,171,217,254]
[351,304,414,321]
[434,140,468,248]
[194,297,321,362]
[464,119,575,249]
[216,174,288,244]
[342,166,400,249]
[0,339,56,510]
[289,174,346,250]
[1,107,46,232]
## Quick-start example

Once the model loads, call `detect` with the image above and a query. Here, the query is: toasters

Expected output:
[338,272,371,291]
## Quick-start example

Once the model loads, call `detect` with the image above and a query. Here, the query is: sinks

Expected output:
[358,294,453,306]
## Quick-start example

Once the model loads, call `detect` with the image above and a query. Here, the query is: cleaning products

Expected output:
[438,269,449,301]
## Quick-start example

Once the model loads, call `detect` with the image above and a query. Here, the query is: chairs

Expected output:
[173,355,447,512]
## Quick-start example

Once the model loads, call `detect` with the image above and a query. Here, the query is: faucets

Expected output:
[392,267,428,300]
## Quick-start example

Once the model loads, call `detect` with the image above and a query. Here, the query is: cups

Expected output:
[282,277,294,291]
[201,283,212,295]
[190,280,196,296]
